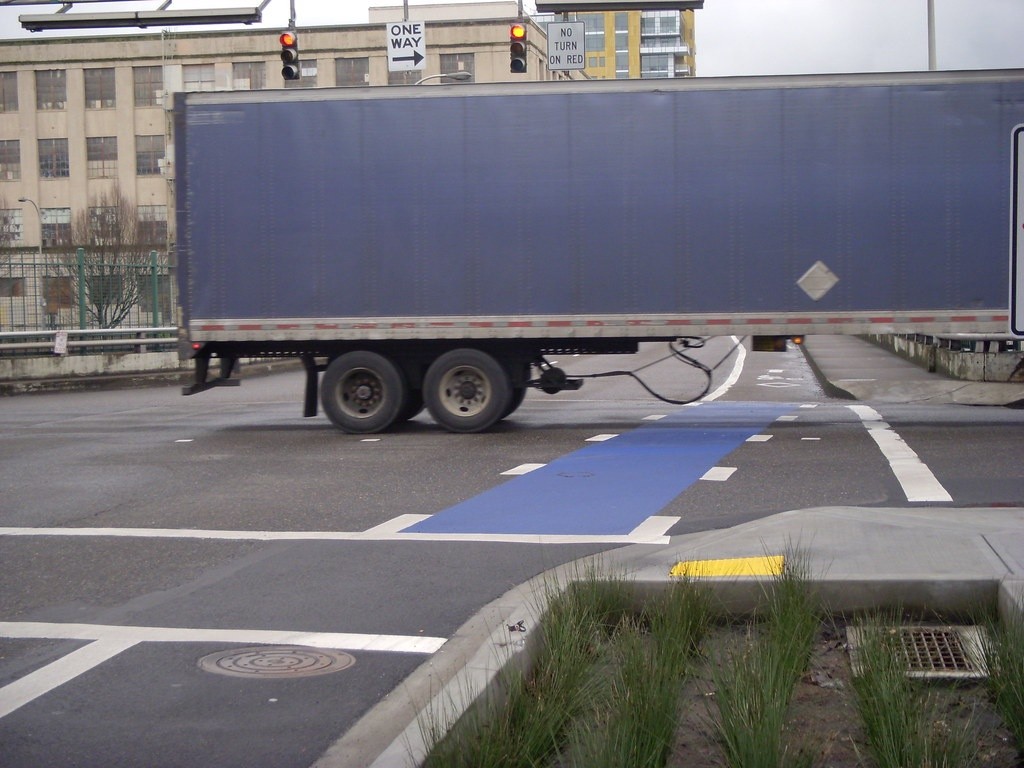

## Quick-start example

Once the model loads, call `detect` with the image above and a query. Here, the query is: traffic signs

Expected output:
[385,21,426,73]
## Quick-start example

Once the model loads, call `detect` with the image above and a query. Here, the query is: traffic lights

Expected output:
[509,23,527,74]
[279,30,302,81]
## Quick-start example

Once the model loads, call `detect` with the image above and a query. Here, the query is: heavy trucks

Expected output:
[167,64,1024,440]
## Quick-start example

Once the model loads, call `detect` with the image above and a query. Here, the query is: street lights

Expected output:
[16,198,46,331]
